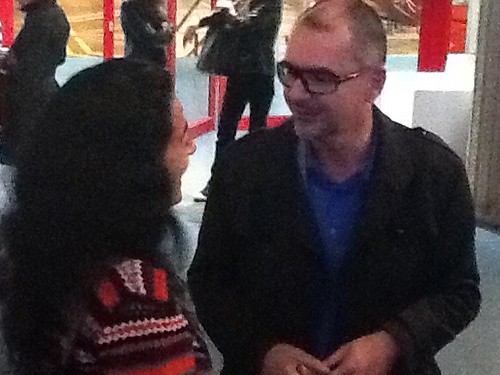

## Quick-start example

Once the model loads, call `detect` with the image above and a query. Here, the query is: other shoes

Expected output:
[193,193,208,202]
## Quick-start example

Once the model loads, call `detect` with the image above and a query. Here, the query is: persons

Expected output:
[187,0,485,375]
[0,60,215,375]
[0,0,175,130]
[184,0,284,203]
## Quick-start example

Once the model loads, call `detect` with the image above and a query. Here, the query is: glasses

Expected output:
[276,60,377,95]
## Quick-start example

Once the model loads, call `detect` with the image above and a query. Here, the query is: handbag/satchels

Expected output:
[196,22,244,75]
